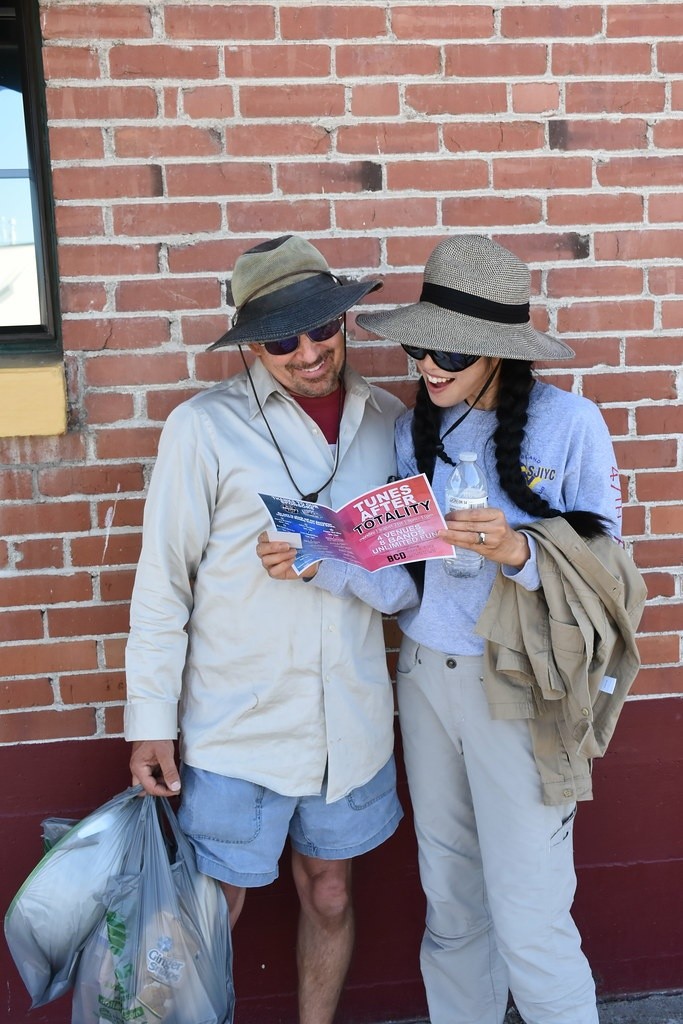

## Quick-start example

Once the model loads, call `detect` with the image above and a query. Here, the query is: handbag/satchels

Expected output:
[4,784,236,1023]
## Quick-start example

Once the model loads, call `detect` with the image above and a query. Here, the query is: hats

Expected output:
[204,235,383,357]
[356,233,578,361]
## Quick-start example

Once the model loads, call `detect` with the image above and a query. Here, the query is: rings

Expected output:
[478,532,486,544]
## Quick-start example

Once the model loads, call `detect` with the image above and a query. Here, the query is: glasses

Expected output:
[258,314,343,355]
[401,343,481,373]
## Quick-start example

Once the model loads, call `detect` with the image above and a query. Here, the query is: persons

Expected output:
[122,232,411,1023]
[258,234,622,1023]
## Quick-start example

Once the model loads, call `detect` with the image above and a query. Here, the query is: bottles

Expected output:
[441,451,489,578]
